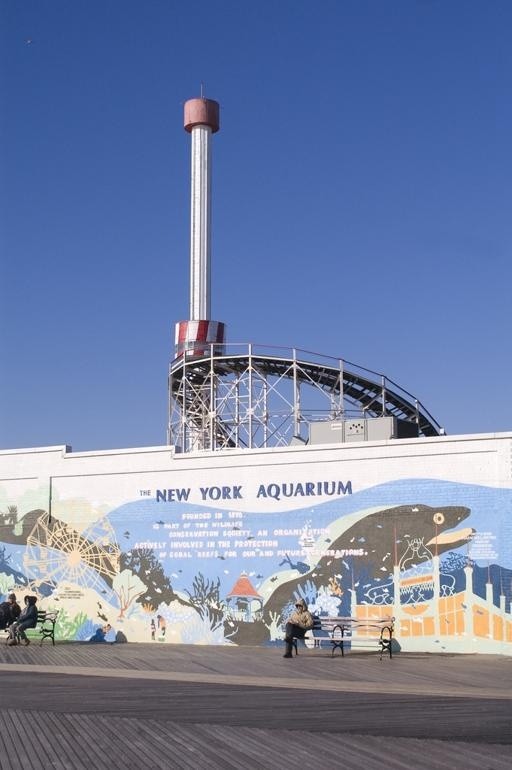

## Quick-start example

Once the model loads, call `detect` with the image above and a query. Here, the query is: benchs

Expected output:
[5,611,59,646]
[291,616,395,661]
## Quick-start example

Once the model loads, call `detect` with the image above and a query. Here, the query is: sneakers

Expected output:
[282,637,295,659]
[7,638,31,646]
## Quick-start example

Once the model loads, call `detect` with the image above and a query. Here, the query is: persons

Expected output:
[157,614,167,641]
[150,618,157,641]
[7,595,37,647]
[282,598,314,658]
[0,592,22,631]
